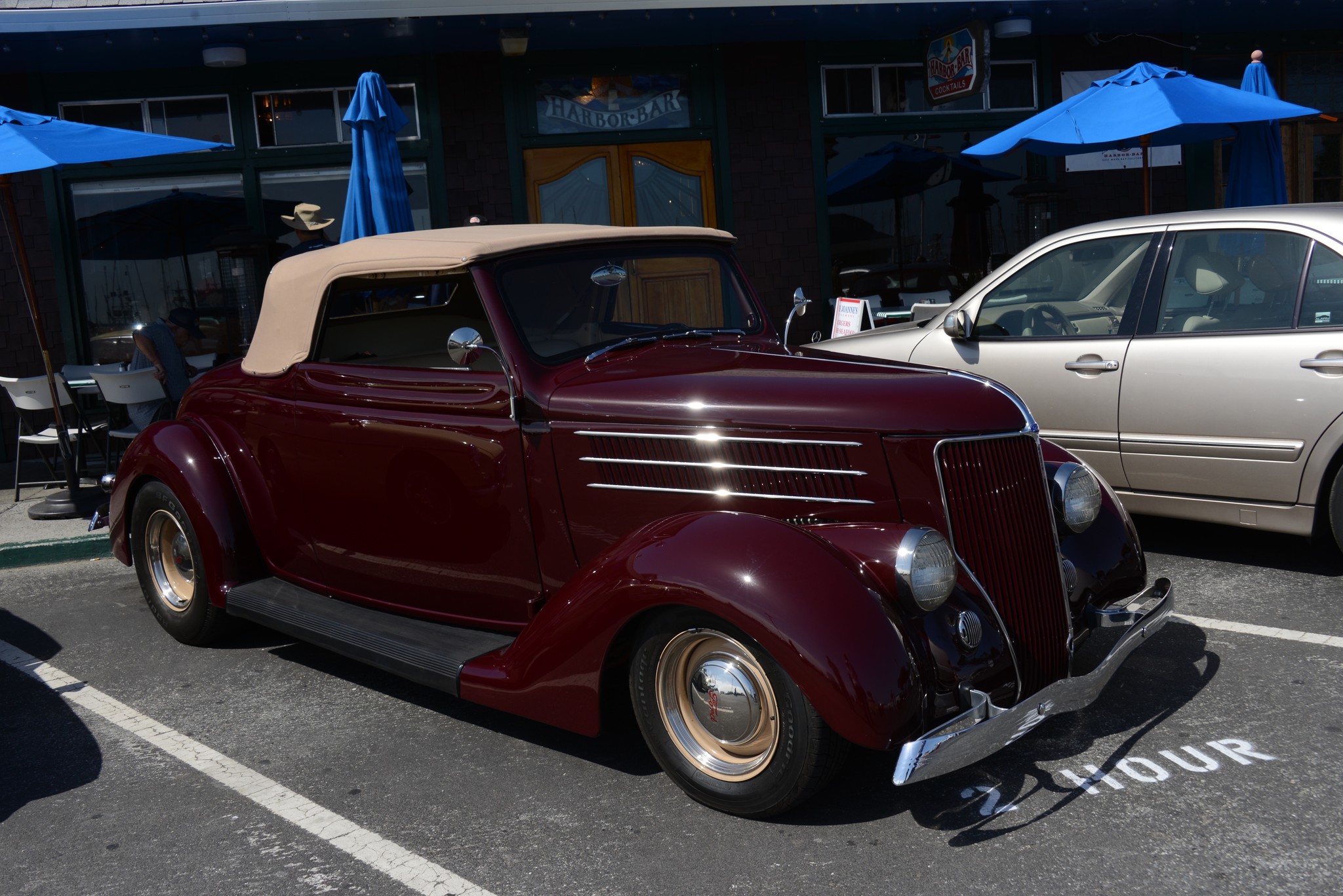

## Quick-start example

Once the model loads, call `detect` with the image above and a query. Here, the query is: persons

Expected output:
[127,307,207,431]
[280,203,339,260]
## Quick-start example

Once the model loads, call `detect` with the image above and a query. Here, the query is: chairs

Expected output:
[1246,254,1294,327]
[1162,252,1249,332]
[0,354,220,502]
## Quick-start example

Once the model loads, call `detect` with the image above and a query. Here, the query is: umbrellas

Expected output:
[1216,50,1289,306]
[825,138,1021,266]
[340,69,415,314]
[0,105,237,520]
[959,61,1323,217]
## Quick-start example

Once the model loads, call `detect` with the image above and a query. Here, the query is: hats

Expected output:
[169,308,206,339]
[280,203,334,230]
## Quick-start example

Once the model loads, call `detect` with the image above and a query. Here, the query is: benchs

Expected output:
[317,313,484,364]
[352,330,611,370]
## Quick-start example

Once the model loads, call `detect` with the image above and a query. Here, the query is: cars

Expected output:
[797,200,1343,575]
[89,215,1174,826]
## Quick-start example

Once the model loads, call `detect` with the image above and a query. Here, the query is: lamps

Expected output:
[497,27,529,57]
[202,47,246,68]
[993,19,1034,38]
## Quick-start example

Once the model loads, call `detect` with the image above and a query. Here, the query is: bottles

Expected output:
[123,291,137,323]
[110,291,123,323]
